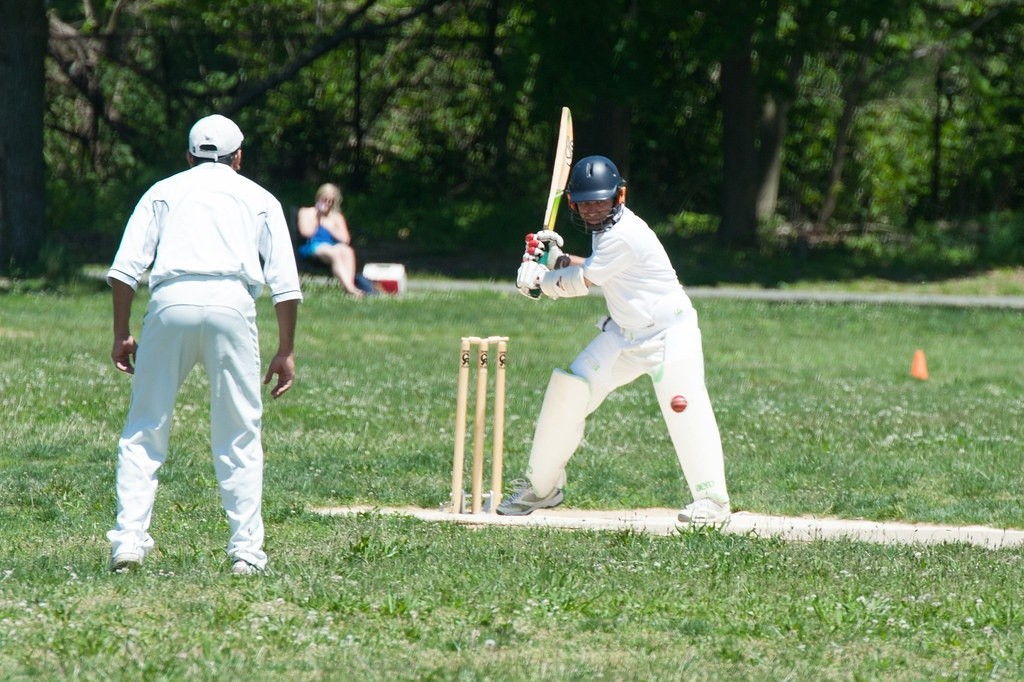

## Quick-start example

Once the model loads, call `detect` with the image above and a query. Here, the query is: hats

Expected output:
[188,114,244,162]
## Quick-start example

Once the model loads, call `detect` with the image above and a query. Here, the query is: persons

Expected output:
[298,183,382,296]
[496,155,731,523]
[107,113,302,577]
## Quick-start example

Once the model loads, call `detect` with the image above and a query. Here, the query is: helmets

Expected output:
[568,156,623,202]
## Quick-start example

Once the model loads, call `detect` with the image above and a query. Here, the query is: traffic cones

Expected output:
[910,349,929,381]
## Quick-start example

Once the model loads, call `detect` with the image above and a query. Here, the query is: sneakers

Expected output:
[496,477,564,516]
[677,495,731,523]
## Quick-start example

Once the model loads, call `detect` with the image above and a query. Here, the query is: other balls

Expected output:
[671,395,688,412]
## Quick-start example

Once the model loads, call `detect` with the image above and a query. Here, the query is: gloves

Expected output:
[515,261,545,300]
[523,229,570,269]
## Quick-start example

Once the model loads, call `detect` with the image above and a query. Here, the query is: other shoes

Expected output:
[231,558,259,576]
[111,553,143,572]
